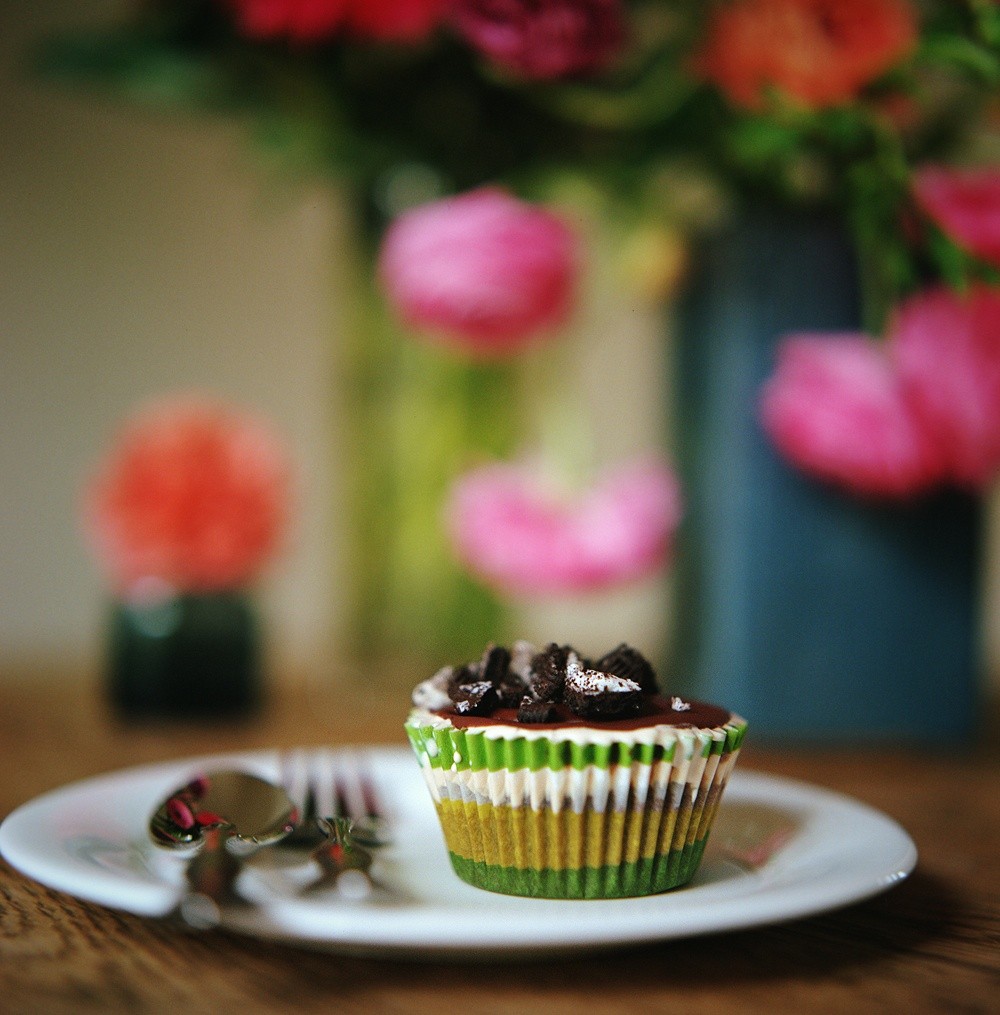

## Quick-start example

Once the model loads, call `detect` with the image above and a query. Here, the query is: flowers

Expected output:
[10,0,1000,585]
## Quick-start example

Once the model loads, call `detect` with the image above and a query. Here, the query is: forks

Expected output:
[273,747,392,901]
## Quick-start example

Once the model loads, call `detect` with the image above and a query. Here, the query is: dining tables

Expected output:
[0,640,1000,1015]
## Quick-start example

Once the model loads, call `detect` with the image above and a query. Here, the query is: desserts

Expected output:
[406,637,749,899]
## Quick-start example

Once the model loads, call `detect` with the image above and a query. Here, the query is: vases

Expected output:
[673,218,981,732]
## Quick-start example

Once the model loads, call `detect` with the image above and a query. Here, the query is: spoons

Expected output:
[147,767,297,932]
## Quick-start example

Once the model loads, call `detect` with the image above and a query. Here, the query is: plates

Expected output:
[0,746,919,949]
